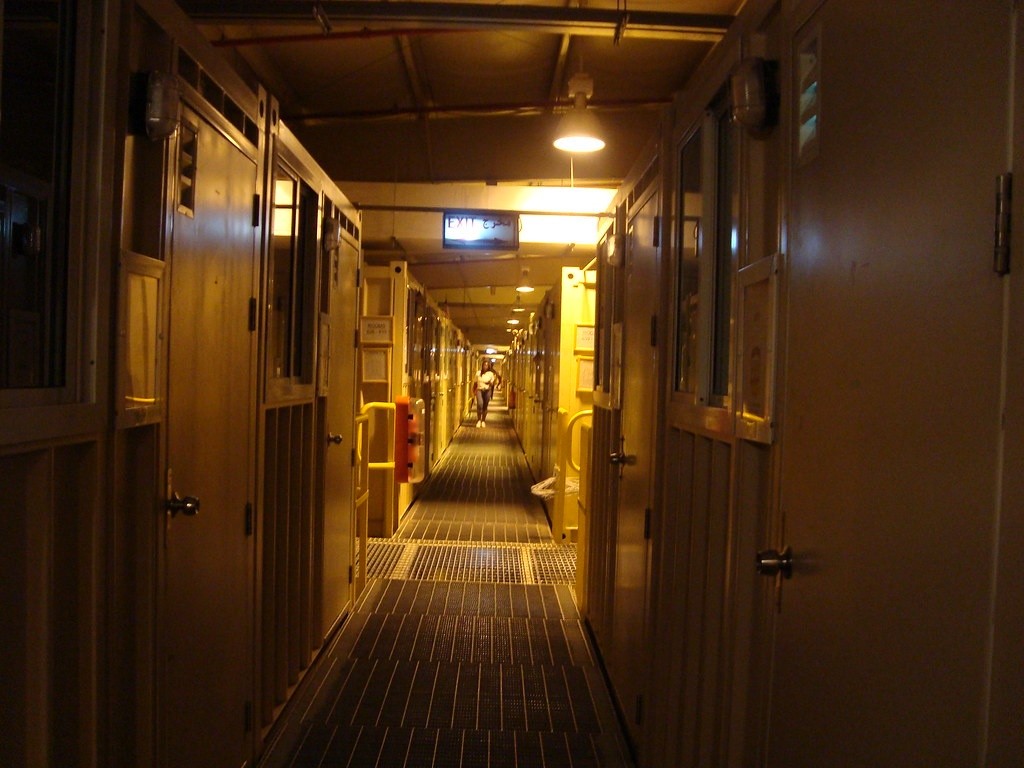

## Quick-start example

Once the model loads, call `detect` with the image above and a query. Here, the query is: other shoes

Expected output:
[476,420,481,428]
[482,422,485,428]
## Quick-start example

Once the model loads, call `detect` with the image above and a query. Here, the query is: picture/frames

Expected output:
[358,315,394,345]
[574,323,594,353]
[576,357,595,393]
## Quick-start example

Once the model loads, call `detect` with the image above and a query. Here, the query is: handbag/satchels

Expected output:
[472,382,477,395]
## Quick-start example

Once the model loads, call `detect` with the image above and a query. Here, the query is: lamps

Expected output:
[516,266,535,293]
[553,54,606,154]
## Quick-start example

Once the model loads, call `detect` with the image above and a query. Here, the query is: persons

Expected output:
[472,360,502,428]
